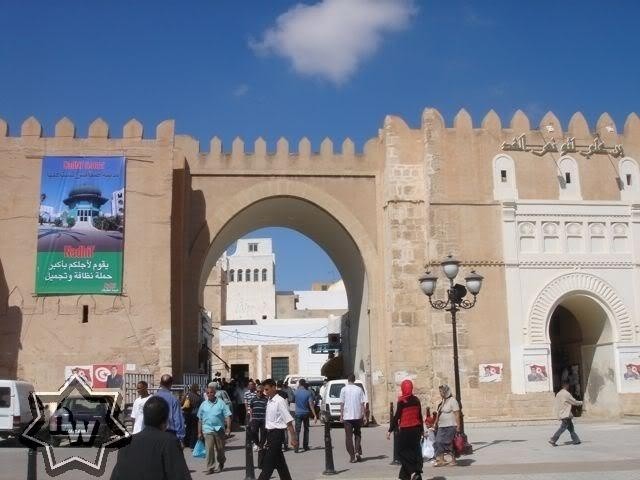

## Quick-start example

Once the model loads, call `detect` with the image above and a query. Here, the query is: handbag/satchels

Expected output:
[454,432,465,454]
[258,444,276,470]
[426,430,435,445]
[181,399,190,410]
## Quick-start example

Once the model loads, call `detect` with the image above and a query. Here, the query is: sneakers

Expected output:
[574,441,581,445]
[549,440,557,446]
[355,453,361,462]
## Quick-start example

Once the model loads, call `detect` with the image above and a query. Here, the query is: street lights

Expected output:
[419,251,485,455]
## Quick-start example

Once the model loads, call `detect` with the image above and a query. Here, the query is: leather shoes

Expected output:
[433,462,447,466]
[447,462,458,466]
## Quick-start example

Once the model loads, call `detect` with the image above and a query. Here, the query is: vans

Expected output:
[0,380,44,447]
[320,379,370,428]
[282,375,327,419]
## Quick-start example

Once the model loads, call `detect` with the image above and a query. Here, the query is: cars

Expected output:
[48,394,125,446]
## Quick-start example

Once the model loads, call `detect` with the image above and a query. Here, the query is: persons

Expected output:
[528,367,542,381]
[106,365,327,480]
[624,365,636,380]
[386,380,423,480]
[339,374,368,463]
[433,384,461,467]
[484,367,492,377]
[548,380,584,446]
